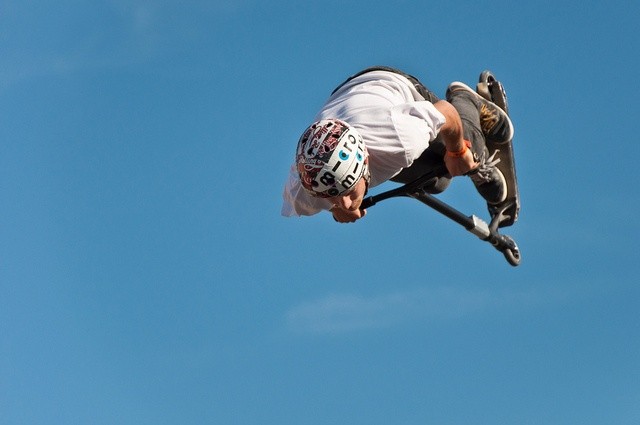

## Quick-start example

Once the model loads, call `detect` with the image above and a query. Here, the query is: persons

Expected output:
[282,67,515,223]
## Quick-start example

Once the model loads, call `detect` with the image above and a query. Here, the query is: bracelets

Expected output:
[443,140,471,162]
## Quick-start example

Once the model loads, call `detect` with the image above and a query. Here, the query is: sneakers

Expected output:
[469,146,507,205]
[446,82,517,145]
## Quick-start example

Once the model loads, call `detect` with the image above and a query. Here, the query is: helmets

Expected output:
[295,118,370,198]
